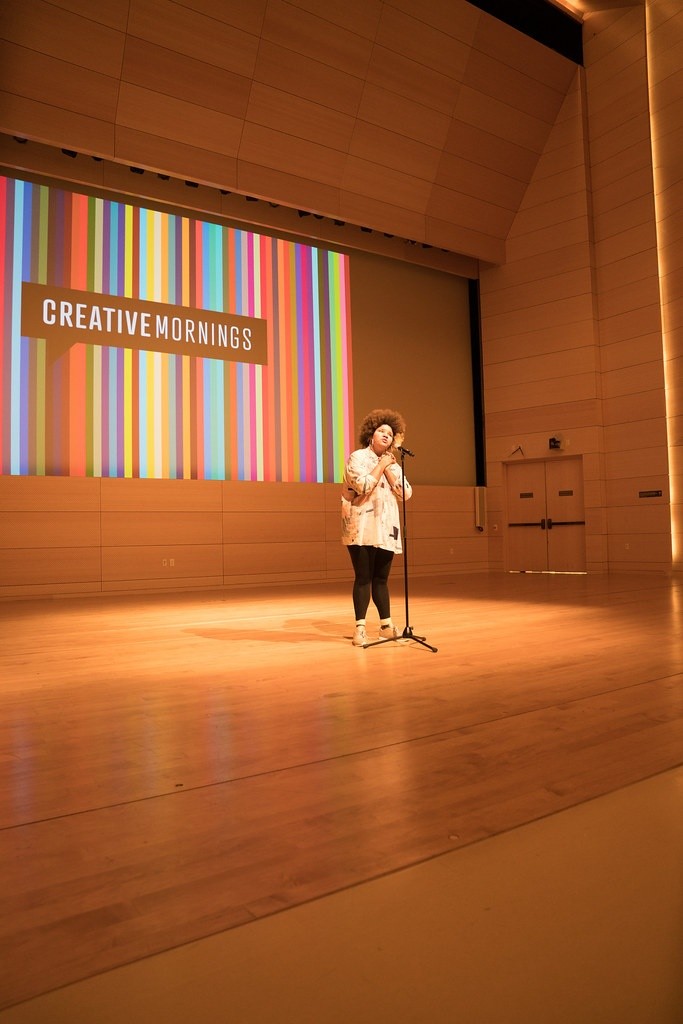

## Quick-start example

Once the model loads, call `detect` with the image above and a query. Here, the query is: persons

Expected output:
[339,408,414,647]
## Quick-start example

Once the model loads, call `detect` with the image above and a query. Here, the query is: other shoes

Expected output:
[352,625,366,648]
[376,625,410,643]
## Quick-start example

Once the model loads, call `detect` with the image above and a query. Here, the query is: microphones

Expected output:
[394,444,415,458]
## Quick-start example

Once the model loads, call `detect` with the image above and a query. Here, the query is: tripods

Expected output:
[363,451,438,654]
